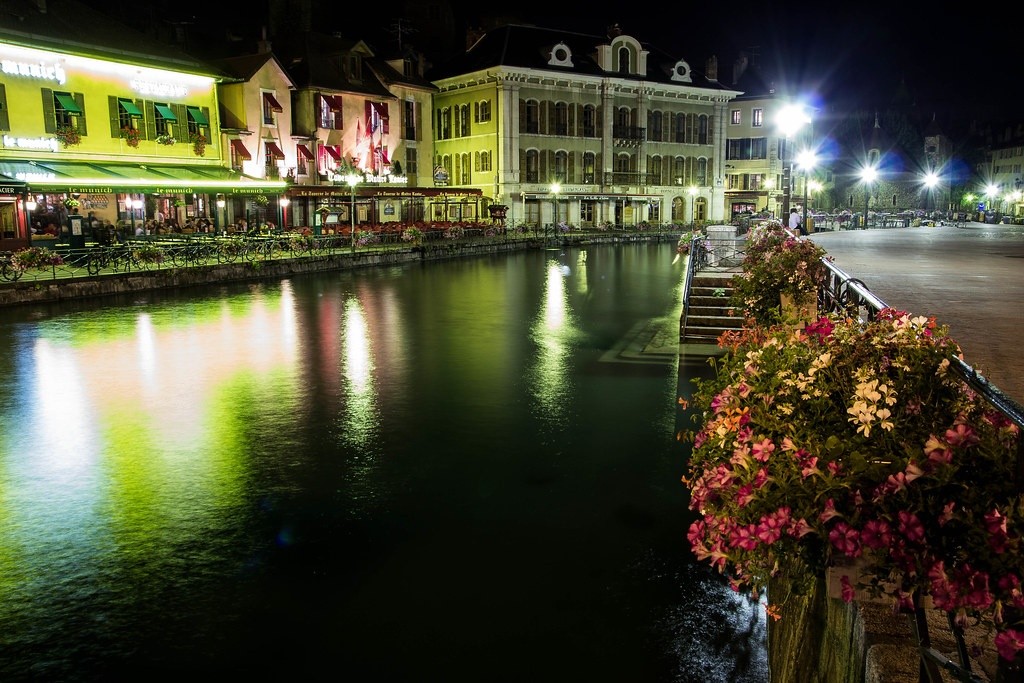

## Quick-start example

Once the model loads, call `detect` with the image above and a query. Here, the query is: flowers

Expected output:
[120,125,140,146]
[190,132,207,156]
[153,134,177,146]
[55,125,80,148]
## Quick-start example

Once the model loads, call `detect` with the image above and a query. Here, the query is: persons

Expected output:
[789,208,810,235]
[68,206,276,236]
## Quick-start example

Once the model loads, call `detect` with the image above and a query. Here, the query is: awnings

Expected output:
[155,104,178,124]
[321,96,341,113]
[323,146,342,162]
[230,139,251,160]
[187,107,210,128]
[119,100,143,120]
[265,142,285,158]
[263,93,283,113]
[55,93,83,116]
[371,104,389,120]
[376,152,390,166]
[298,144,315,160]
[0,161,285,246]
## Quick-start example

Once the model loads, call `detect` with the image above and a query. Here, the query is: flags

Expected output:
[355,121,361,146]
[373,125,381,149]
[363,120,371,142]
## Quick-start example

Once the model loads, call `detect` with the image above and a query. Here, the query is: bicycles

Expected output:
[86,233,337,274]
[0,251,23,282]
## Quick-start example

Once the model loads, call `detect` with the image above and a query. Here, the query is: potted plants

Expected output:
[172,199,185,206]
[65,198,79,209]
[251,194,268,207]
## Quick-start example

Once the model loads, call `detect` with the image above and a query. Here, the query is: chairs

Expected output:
[284,218,491,247]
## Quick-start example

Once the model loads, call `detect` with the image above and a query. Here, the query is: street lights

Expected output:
[764,180,773,210]
[986,185,997,209]
[775,103,804,227]
[797,150,815,235]
[1005,191,1021,223]
[861,168,875,229]
[688,186,698,222]
[551,183,562,234]
[347,174,358,253]
[925,174,936,224]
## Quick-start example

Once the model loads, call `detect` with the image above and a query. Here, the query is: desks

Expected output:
[55,231,300,267]
